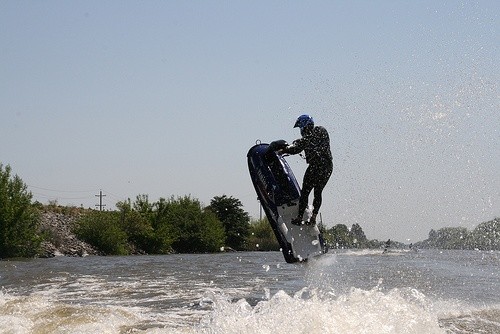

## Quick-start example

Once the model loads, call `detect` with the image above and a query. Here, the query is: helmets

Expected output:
[293,115,314,134]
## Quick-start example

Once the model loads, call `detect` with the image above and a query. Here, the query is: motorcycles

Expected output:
[246,139,329,264]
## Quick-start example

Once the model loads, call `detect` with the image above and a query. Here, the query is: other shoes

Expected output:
[305,219,316,227]
[291,218,301,226]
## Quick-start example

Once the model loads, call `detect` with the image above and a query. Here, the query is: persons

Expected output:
[382,239,391,254]
[277,114,334,228]
